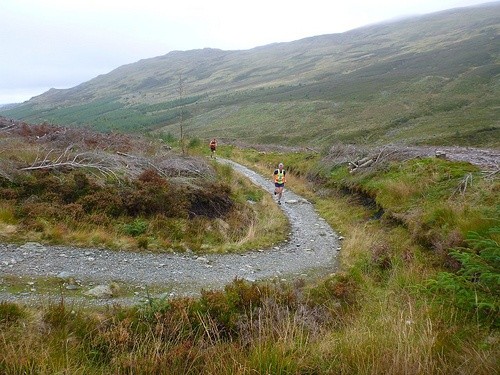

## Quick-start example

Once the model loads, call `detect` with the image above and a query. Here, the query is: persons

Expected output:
[209,137,218,160]
[272,163,287,203]
